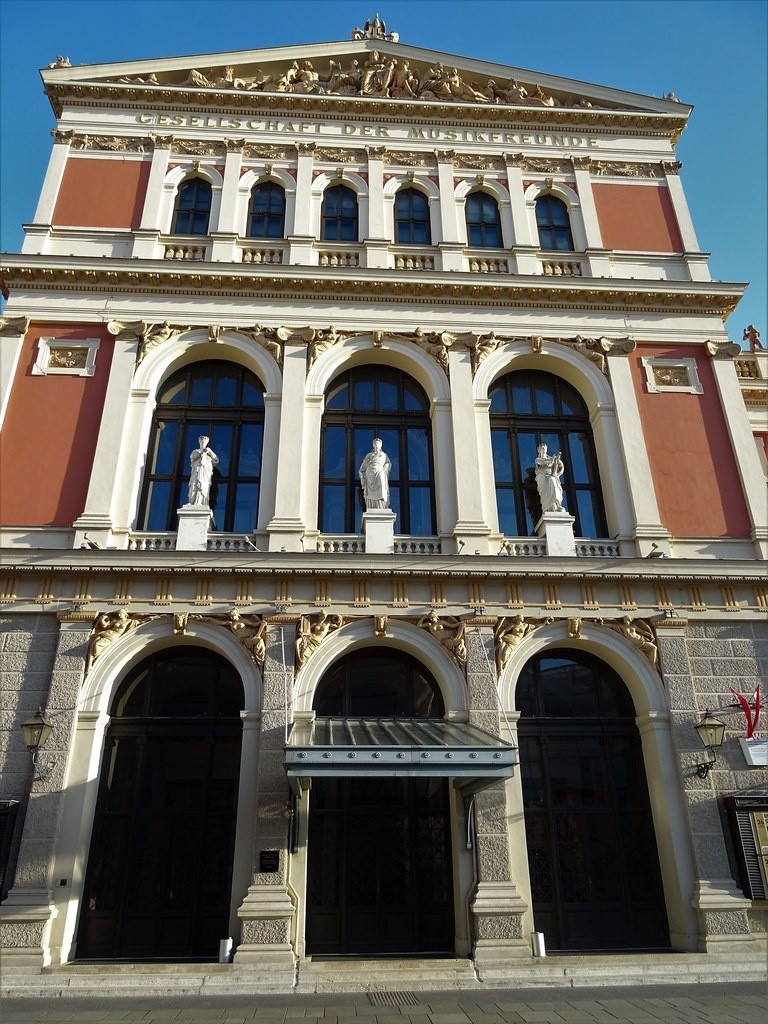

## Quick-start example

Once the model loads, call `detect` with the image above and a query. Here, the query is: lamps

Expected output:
[21,707,54,781]
[695,708,726,778]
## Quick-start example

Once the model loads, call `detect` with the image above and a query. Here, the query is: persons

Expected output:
[595,614,657,667]
[49,56,70,67]
[391,327,449,375]
[415,610,466,669]
[196,607,266,668]
[189,436,219,505]
[535,444,565,511]
[297,608,342,670]
[136,320,192,364]
[358,438,392,508]
[556,335,607,373]
[353,13,399,41]
[84,608,153,674]
[497,613,554,673]
[307,326,361,370]
[118,50,625,110]
[474,331,516,373]
[235,323,284,364]
[663,91,680,102]
[743,325,764,350]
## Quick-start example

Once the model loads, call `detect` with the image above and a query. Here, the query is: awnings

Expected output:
[283,716,518,853]
